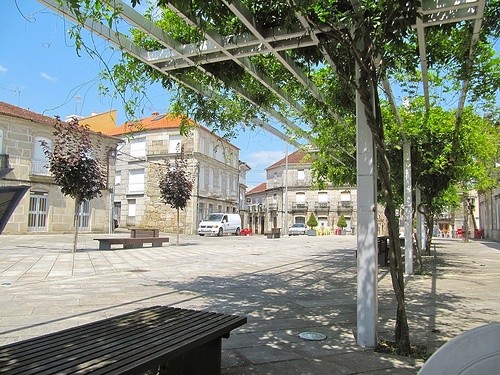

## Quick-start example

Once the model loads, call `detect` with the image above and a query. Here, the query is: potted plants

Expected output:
[306,213,318,236]
[337,212,347,235]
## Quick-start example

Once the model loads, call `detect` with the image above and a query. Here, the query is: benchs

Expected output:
[0,306,248,374]
[92,237,170,251]
[355,237,389,266]
[265,227,281,239]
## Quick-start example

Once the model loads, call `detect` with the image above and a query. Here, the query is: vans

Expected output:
[198,212,241,237]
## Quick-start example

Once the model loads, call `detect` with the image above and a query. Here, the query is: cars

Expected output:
[287,222,307,236]
